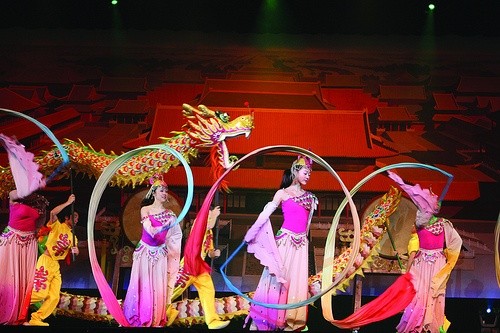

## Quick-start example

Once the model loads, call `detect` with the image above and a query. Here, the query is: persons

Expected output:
[243,156,318,331]
[395,214,462,333]
[0,191,49,326]
[121,175,183,327]
[26,194,78,326]
[167,205,231,330]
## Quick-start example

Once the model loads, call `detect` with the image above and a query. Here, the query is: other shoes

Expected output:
[208,320,230,329]
[250,322,257,331]
[22,320,49,326]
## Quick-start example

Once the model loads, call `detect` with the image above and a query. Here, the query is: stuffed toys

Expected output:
[1,103,401,328]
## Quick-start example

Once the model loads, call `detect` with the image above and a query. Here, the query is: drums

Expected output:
[361,194,419,259]
[119,187,189,250]
